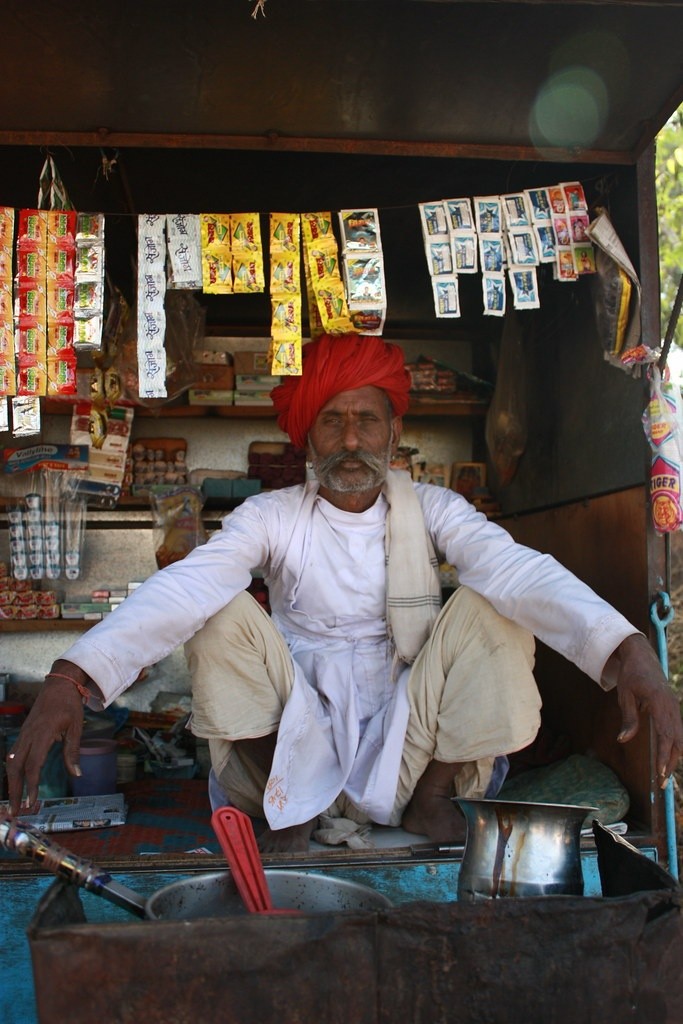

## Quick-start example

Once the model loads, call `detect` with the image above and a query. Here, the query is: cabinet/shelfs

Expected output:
[0,319,494,515]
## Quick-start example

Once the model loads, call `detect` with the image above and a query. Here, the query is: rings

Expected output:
[9,753,15,759]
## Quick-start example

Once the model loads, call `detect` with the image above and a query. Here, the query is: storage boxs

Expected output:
[233,479,261,498]
[192,350,233,366]
[236,375,282,391]
[189,389,234,406]
[0,560,144,620]
[204,478,233,498]
[234,390,274,406]
[233,350,271,374]
[188,365,235,391]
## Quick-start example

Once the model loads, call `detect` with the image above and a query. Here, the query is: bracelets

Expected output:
[46,674,90,705]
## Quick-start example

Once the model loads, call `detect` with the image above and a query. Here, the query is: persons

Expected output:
[6,333,683,858]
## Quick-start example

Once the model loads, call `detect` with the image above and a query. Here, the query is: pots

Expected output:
[0,815,393,921]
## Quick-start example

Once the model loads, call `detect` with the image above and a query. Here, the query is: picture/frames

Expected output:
[453,462,487,499]
